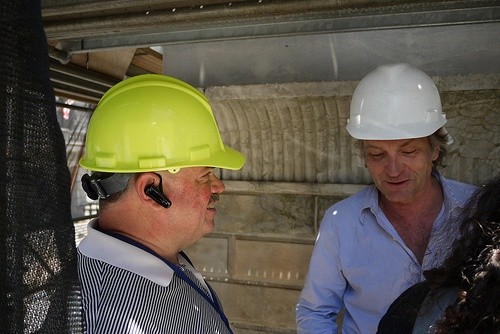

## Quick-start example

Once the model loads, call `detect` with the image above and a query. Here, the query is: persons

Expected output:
[376,172,500,334]
[38,74,248,334]
[294,62,483,334]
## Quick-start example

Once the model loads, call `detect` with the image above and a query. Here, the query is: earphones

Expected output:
[145,184,171,209]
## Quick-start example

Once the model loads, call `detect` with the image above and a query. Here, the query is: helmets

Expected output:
[346,62,448,140]
[78,73,246,174]
